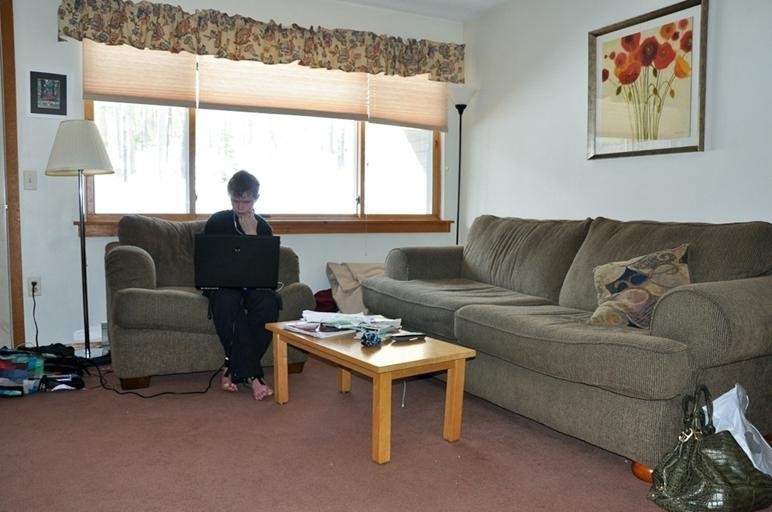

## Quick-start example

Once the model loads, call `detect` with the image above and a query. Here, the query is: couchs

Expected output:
[359,215,772,483]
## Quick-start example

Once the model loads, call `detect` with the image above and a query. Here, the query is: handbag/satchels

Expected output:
[645,384,772,512]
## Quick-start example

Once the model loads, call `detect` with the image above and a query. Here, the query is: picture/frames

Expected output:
[586,0,708,161]
[28,70,68,116]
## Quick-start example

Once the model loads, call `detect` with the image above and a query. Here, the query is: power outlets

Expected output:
[28,276,42,296]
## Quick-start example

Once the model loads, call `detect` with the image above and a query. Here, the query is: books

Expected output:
[283,309,403,340]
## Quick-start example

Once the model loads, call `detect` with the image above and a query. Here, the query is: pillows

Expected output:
[588,242,692,332]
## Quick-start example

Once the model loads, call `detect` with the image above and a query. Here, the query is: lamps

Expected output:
[46,120,117,366]
[446,85,478,244]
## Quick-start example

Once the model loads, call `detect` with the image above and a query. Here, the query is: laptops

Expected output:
[194,234,280,290]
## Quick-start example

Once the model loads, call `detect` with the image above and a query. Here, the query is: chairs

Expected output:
[103,214,317,391]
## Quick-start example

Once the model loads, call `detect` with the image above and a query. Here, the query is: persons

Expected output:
[201,170,283,401]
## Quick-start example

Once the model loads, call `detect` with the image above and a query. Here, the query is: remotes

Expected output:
[390,333,426,341]
